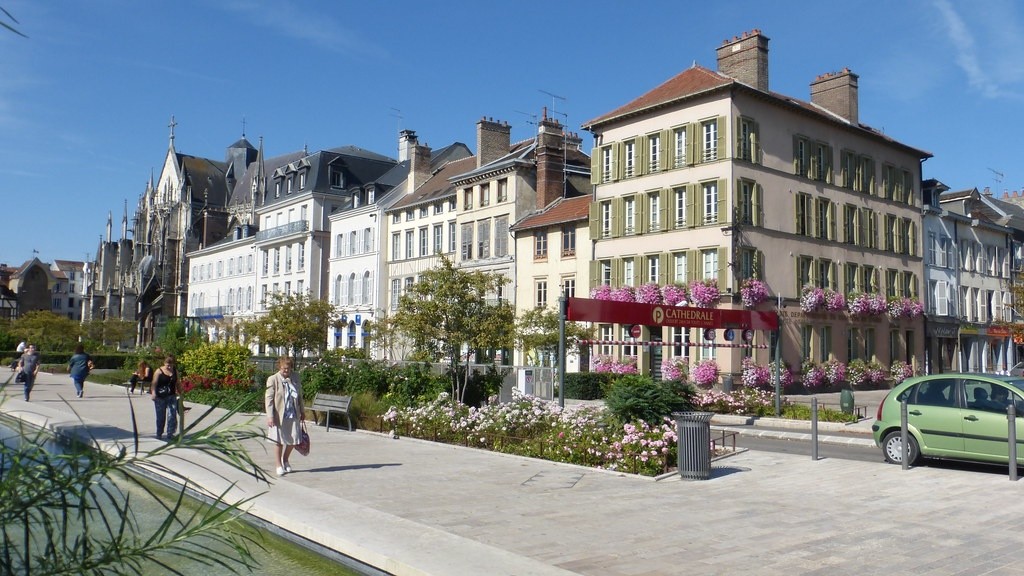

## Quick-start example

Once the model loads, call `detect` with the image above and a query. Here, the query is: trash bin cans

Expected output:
[722,373,735,396]
[518,368,536,398]
[672,411,716,482]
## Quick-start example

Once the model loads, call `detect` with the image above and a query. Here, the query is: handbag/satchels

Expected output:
[87,359,95,371]
[16,368,26,383]
[156,383,175,397]
[293,420,310,457]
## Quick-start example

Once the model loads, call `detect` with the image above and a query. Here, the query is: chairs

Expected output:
[974,387,990,407]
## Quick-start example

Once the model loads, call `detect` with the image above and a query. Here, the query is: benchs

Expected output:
[122,378,147,395]
[302,393,352,431]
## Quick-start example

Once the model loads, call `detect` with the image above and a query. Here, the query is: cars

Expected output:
[873,374,1024,468]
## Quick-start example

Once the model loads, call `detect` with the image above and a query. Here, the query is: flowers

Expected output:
[803,358,885,387]
[891,361,913,385]
[742,278,769,308]
[661,356,718,388]
[590,277,720,309]
[741,357,794,388]
[591,354,636,374]
[800,283,923,321]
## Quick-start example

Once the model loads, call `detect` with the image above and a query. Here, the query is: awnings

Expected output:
[168,314,223,319]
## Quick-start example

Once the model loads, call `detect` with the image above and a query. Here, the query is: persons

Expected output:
[123,360,152,395]
[265,357,305,474]
[18,344,40,401]
[67,342,92,398]
[990,388,1008,412]
[8,349,29,371]
[152,357,179,440]
[17,340,25,352]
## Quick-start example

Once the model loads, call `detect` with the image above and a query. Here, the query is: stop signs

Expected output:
[631,325,641,338]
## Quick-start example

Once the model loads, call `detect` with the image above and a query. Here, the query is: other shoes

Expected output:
[76,390,83,398]
[25,398,28,401]
[166,434,178,441]
[123,382,131,385]
[125,392,133,395]
[156,434,163,440]
[276,463,292,475]
